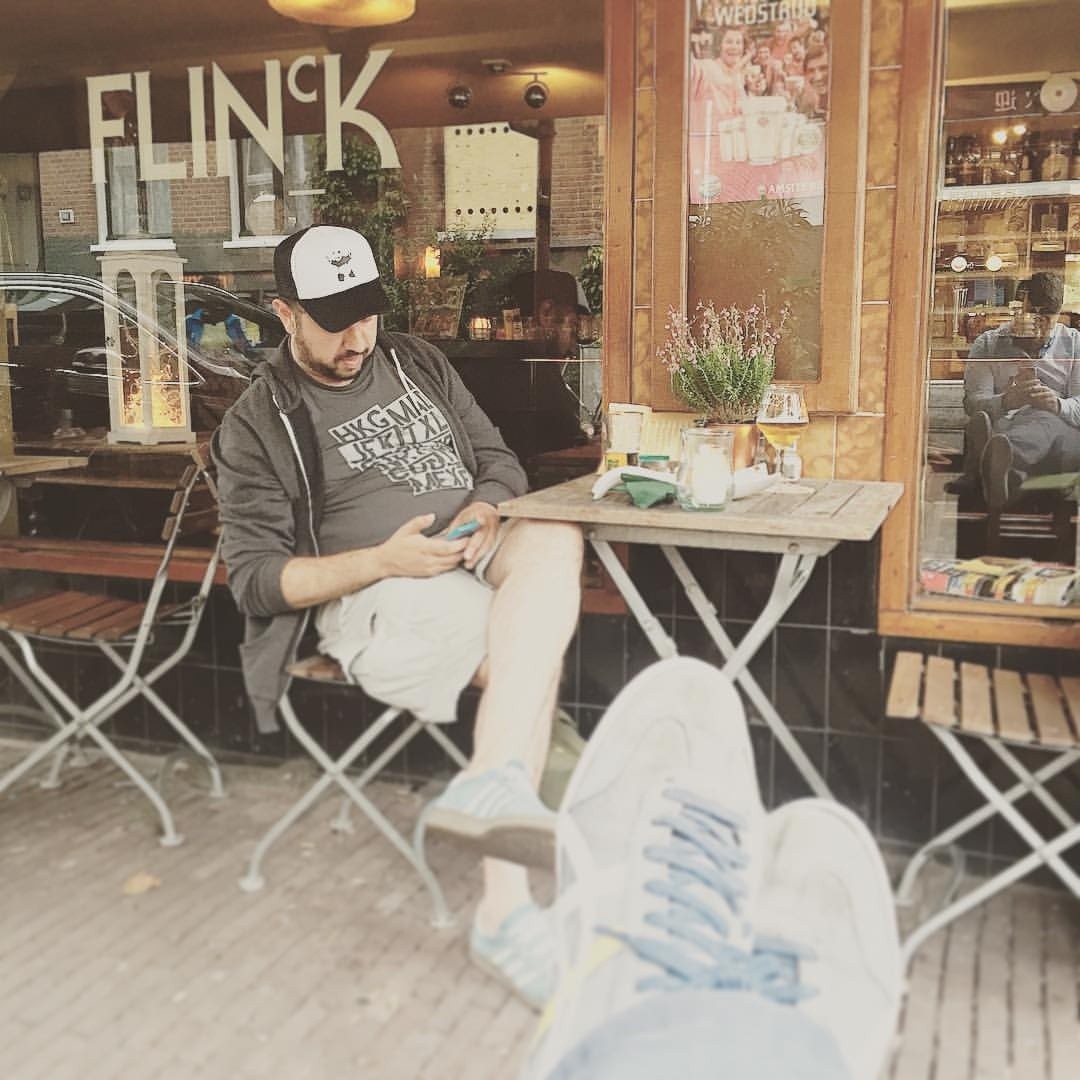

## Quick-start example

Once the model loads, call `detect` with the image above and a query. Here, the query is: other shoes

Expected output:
[421,761,558,874]
[964,411,1013,507]
[470,901,567,1014]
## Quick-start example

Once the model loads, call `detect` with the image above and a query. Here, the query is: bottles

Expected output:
[639,455,670,473]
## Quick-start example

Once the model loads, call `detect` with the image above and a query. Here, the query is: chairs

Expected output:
[960,309,1080,566]
[886,651,1080,978]
[192,445,567,932]
[0,449,224,847]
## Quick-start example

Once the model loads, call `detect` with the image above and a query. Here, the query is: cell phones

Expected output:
[448,521,479,542]
[1016,367,1037,383]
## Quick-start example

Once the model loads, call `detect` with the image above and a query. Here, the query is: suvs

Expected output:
[0,272,252,444]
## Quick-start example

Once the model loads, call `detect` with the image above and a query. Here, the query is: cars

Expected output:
[95,277,287,375]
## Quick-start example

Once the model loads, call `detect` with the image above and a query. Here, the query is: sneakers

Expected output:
[519,657,908,1080]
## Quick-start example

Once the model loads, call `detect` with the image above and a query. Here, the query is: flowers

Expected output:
[656,288,790,421]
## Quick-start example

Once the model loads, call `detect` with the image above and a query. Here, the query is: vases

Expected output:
[693,420,759,470]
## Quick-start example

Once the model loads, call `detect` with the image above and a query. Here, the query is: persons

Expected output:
[211,220,596,836]
[962,270,1080,512]
[537,651,902,1080]
[184,277,257,356]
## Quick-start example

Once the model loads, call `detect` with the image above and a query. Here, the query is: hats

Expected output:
[514,270,589,315]
[1017,271,1063,313]
[272,224,391,333]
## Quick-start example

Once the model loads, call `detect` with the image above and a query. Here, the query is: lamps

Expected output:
[447,59,550,109]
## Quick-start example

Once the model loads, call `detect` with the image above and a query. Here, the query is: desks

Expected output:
[498,477,903,804]
[0,455,89,525]
[429,339,578,494]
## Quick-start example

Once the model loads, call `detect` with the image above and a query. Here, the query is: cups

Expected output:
[674,428,735,512]
